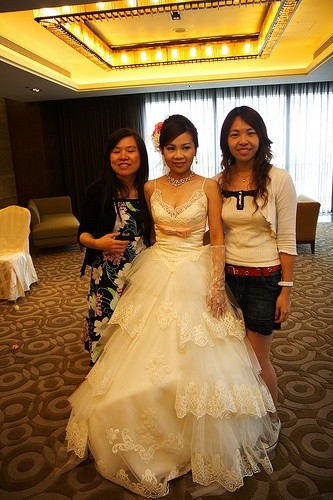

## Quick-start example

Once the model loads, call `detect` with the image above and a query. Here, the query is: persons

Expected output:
[203,105,298,454]
[77,129,151,367]
[66,114,275,499]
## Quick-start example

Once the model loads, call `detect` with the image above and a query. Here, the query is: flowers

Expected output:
[152,122,163,152]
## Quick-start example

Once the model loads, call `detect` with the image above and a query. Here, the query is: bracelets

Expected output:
[278,281,294,287]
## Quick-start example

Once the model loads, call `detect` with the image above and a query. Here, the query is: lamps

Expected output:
[33,0,302,72]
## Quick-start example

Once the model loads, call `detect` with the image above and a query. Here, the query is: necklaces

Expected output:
[237,174,252,182]
[165,171,195,187]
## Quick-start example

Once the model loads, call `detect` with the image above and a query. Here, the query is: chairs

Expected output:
[28,196,80,256]
[0,205,39,301]
[296,195,321,254]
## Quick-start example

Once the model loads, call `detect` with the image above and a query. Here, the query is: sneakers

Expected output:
[259,417,282,452]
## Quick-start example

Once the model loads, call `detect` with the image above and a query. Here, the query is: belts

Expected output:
[223,263,281,278]
